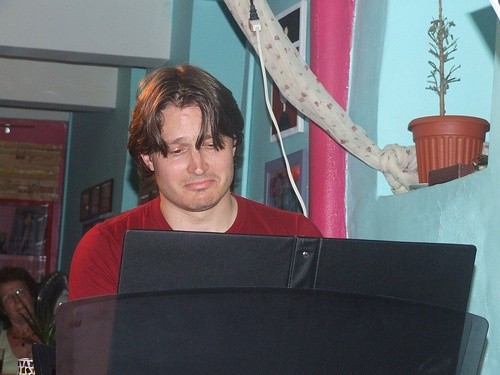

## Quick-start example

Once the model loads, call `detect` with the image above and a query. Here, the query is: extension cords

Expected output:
[249,19,261,31]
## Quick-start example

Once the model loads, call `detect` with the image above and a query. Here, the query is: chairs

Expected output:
[53,288,489,375]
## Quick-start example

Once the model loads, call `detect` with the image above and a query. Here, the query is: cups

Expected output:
[0,349,5,373]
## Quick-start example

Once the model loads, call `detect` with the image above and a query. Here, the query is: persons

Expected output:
[0,266,44,375]
[67,63,326,301]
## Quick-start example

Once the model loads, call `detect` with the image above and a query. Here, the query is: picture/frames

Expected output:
[80,178,113,221]
[269,0,308,143]
[263,148,305,214]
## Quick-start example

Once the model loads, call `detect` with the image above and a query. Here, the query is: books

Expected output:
[6,205,48,256]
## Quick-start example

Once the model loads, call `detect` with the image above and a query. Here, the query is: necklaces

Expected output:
[13,329,29,347]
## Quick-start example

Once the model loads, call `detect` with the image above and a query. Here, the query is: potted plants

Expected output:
[408,0,491,183]
[14,291,59,375]
